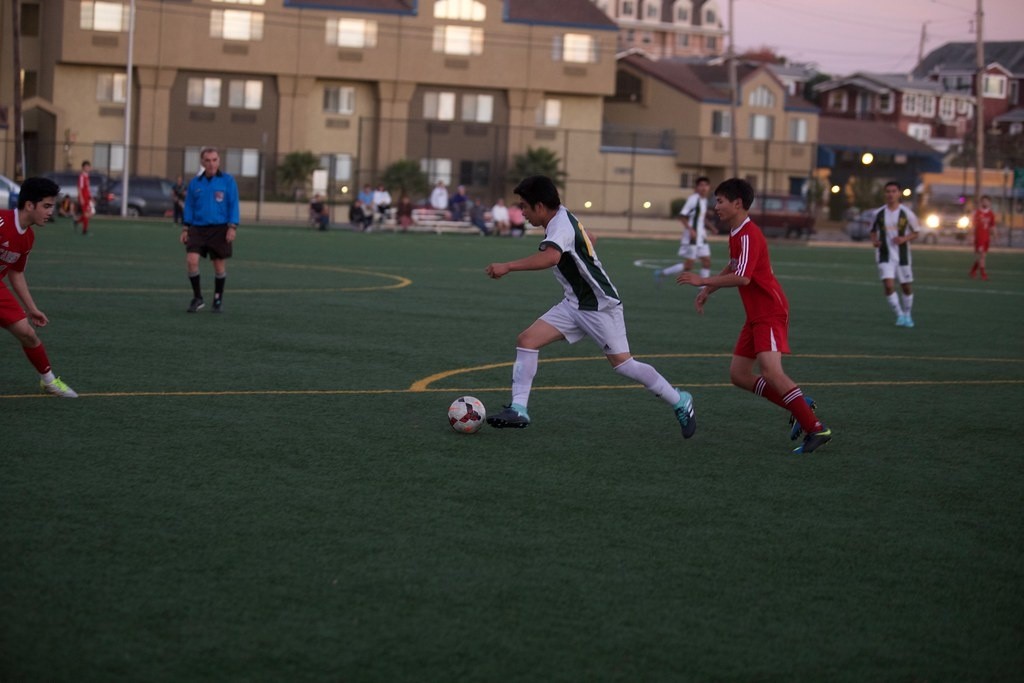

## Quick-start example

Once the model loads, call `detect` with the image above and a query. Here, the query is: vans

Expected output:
[705,192,816,239]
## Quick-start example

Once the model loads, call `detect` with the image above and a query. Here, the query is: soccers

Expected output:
[448,396,487,434]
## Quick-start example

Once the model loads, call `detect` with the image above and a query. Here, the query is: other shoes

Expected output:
[968,271,978,279]
[903,315,915,328]
[980,273,989,280]
[653,270,665,290]
[895,316,905,327]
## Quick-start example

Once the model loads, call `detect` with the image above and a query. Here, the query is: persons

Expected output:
[870,181,920,328]
[470,197,491,236]
[172,174,188,224]
[72,161,97,236]
[99,177,109,215]
[429,180,449,209]
[349,182,378,233]
[0,176,80,398]
[968,195,996,281]
[308,193,329,231]
[397,195,413,233]
[676,176,833,455]
[655,177,719,290]
[58,193,77,220]
[506,199,526,237]
[374,183,392,222]
[449,184,468,221]
[485,175,697,439]
[492,197,511,236]
[179,147,240,314]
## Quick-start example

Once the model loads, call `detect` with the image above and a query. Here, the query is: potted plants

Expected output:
[374,159,431,206]
[273,151,326,203]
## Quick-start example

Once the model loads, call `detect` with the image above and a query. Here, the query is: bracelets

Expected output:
[182,229,188,233]
[227,225,237,230]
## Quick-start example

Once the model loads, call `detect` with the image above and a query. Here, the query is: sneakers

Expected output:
[187,296,205,312]
[792,426,834,455]
[212,293,223,312]
[789,398,817,440]
[40,376,79,398]
[485,403,529,429]
[671,387,697,438]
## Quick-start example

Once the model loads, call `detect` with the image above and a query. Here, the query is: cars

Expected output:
[840,200,972,243]
[0,168,183,219]
